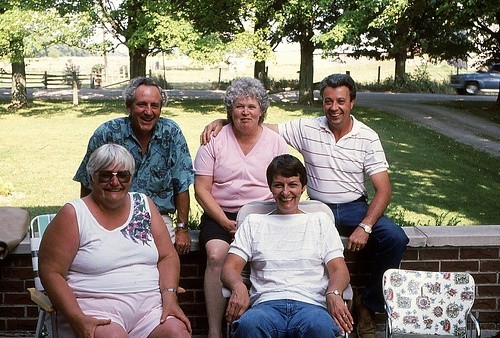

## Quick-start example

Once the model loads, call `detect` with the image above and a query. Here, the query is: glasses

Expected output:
[94,169,132,183]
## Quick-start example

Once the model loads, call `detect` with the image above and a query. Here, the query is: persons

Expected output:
[72,76,194,256]
[193,76,291,338]
[200,73,409,338]
[38,143,192,338]
[220,154,354,338]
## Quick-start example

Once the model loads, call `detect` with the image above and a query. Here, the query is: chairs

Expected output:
[221,200,353,338]
[382,269,481,338]
[26,214,186,338]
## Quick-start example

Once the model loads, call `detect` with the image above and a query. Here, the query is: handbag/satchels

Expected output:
[0,207,30,260]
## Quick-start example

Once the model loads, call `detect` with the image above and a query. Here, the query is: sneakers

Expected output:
[355,295,375,338]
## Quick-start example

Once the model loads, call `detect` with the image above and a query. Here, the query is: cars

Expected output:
[448,62,500,96]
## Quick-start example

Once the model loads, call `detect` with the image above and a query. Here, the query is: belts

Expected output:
[159,211,169,215]
[354,196,367,202]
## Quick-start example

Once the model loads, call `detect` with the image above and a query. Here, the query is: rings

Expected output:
[342,313,345,317]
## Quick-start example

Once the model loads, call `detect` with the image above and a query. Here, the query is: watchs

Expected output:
[359,223,372,233]
[176,222,188,228]
[326,290,341,296]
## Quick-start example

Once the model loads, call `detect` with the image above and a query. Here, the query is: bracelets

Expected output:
[162,287,177,293]
